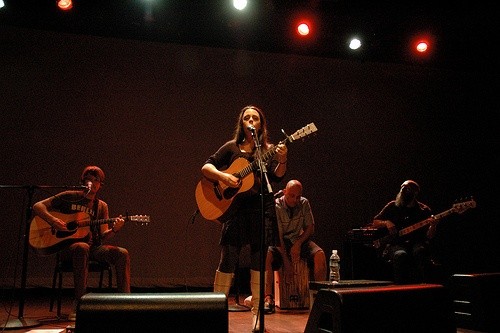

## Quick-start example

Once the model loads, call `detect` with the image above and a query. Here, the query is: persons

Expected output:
[372,180,436,285]
[264,180,327,312]
[202,105,288,332]
[33,166,131,302]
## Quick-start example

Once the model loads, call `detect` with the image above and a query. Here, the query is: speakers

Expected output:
[452,273,500,333]
[75,292,229,333]
[303,283,456,333]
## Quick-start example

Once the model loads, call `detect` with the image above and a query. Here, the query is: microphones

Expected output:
[88,181,92,190]
[247,124,256,130]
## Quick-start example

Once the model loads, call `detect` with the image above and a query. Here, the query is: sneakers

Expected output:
[264,295,275,313]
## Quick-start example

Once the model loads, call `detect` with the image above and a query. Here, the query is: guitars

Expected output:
[28,210,151,253]
[364,194,477,255]
[195,122,318,222]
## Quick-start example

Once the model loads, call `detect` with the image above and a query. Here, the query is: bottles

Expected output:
[329,250,341,282]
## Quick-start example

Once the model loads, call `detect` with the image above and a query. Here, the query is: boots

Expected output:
[214,270,234,297]
[250,269,269,331]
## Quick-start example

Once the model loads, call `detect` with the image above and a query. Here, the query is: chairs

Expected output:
[49,256,112,317]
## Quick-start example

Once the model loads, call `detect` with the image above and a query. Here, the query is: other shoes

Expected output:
[68,311,79,321]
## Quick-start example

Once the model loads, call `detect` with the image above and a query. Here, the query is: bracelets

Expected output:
[112,229,119,233]
[279,159,287,164]
